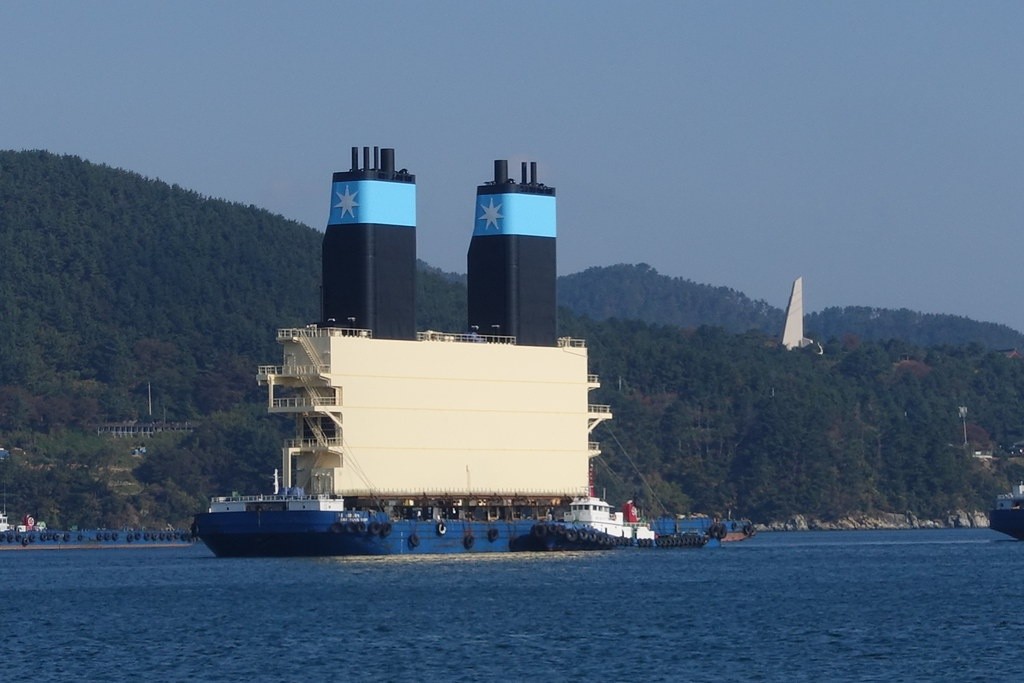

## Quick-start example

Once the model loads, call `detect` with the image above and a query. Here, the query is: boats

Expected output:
[987,482,1024,538]
[183,146,756,564]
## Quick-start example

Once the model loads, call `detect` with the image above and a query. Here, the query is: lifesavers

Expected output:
[534,522,653,548]
[709,525,726,538]
[438,523,447,535]
[127,530,193,543]
[656,536,707,547]
[331,522,392,537]
[464,535,474,547]
[410,534,420,546]
[97,532,118,540]
[4,532,70,545]
[488,527,499,540]
[191,523,200,537]
[745,525,756,536]
[732,522,737,529]
[78,535,82,540]
